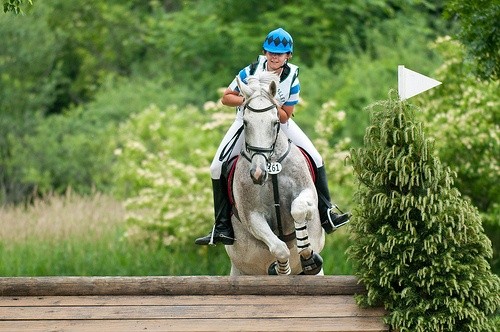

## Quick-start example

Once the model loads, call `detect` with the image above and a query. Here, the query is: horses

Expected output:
[223,70,325,276]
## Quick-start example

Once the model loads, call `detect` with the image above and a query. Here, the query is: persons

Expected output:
[193,28,353,245]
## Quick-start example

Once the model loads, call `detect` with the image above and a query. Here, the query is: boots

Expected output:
[195,177,234,246]
[313,164,352,235]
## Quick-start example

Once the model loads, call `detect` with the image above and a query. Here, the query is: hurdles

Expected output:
[0,275,390,332]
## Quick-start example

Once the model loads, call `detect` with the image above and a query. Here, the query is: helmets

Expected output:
[263,28,294,55]
